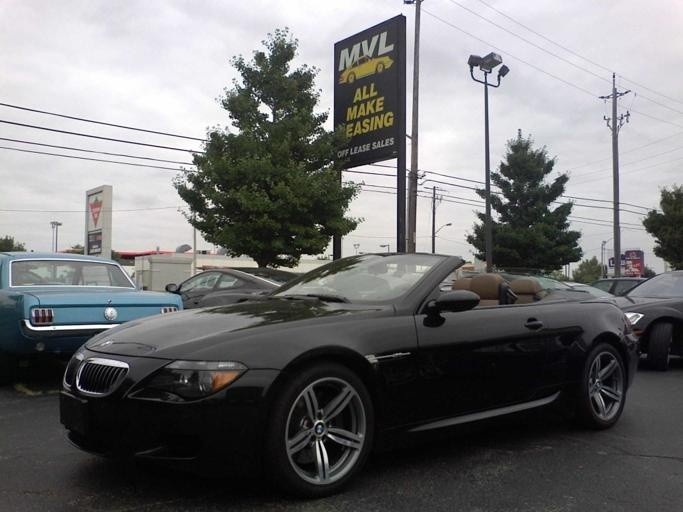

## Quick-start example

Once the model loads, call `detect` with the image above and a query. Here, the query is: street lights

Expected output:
[432,223,453,253]
[379,244,390,252]
[49,220,62,253]
[464,50,510,271]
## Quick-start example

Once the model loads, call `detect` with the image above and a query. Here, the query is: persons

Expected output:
[626,261,640,272]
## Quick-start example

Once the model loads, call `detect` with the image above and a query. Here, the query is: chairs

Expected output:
[446,273,540,308]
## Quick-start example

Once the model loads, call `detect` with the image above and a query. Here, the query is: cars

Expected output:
[526,271,651,304]
[613,268,682,372]
[0,250,187,375]
[163,265,303,310]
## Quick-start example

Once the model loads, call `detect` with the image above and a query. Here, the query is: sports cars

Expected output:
[60,253,638,502]
